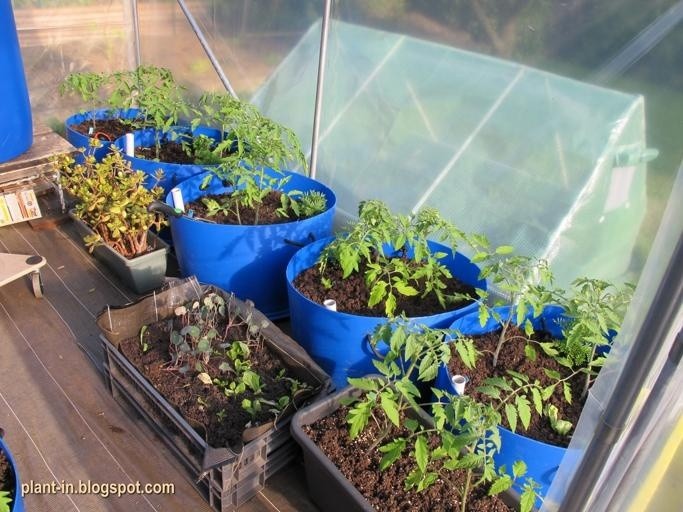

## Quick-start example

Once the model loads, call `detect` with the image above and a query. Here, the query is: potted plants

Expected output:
[52,65,640,512]
[0,440,26,511]
